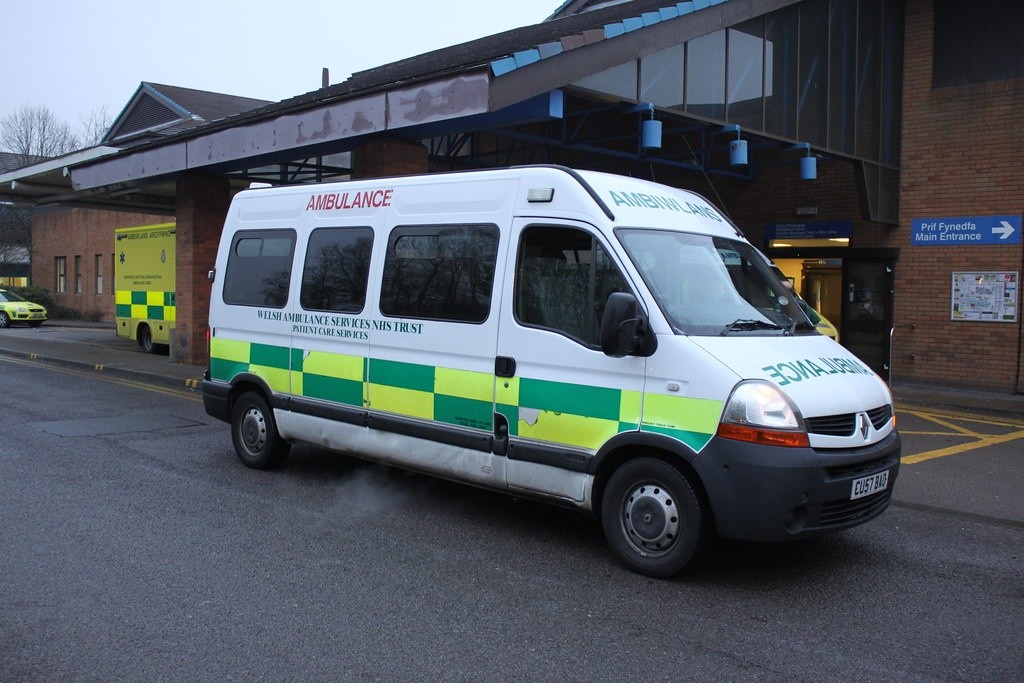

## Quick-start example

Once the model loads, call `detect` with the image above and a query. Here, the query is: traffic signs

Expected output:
[911,215,1022,248]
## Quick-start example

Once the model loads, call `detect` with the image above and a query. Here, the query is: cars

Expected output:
[0,288,49,328]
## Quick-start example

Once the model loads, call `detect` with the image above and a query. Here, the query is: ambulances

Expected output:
[114,220,178,353]
[199,160,905,579]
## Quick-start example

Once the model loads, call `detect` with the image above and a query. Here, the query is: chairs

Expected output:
[524,248,601,346]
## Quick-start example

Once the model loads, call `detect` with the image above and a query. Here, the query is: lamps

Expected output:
[729,124,748,166]
[644,102,661,149]
[801,142,818,180]
